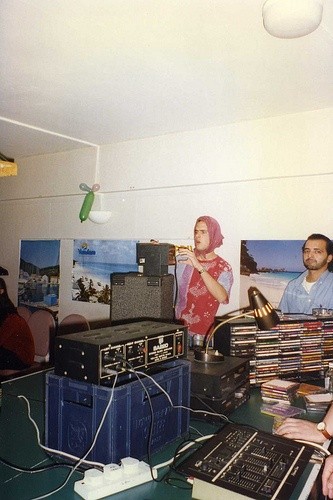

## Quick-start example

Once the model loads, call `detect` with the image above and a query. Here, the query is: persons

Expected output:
[276,234,333,314]
[0,277,34,370]
[152,216,233,348]
[276,402,333,499]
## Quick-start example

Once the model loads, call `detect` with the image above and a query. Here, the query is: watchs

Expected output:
[316,422,332,439]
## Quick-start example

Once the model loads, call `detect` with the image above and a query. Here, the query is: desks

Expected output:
[0,365,332,500]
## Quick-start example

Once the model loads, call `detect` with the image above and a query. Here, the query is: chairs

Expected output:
[15,305,91,367]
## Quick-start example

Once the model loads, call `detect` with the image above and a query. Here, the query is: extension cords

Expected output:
[74,461,158,500]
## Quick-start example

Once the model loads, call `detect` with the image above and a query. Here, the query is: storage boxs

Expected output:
[44,358,192,468]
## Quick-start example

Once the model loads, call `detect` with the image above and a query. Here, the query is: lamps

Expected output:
[194,286,281,363]
[262,0,323,38]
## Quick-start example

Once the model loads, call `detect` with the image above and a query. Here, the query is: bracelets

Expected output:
[198,268,205,274]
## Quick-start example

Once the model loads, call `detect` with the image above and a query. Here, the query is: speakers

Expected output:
[109,273,175,321]
